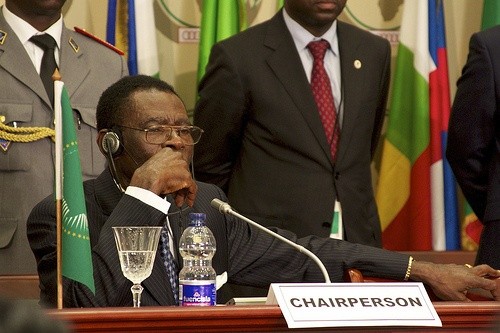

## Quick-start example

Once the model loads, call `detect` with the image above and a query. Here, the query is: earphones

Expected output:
[103,132,119,153]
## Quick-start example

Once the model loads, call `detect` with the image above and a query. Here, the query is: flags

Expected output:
[376,0,461,254]
[50,80,96,297]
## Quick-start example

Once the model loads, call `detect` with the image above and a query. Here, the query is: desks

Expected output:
[46,300,500,333]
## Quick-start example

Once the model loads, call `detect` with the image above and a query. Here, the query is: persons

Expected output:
[446,24,500,269]
[25,74,500,310]
[192,1,393,279]
[0,0,125,333]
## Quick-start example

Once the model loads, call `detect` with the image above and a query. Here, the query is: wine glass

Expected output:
[112,225,164,308]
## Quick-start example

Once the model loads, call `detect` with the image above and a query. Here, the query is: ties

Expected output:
[29,33,59,109]
[306,39,341,162]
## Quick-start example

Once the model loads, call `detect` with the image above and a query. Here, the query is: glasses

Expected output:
[113,122,204,146]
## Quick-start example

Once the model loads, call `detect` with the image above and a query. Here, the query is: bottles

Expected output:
[177,212,217,307]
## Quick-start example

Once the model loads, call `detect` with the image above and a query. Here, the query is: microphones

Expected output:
[210,198,331,283]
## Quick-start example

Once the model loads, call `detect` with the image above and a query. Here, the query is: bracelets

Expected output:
[404,256,415,282]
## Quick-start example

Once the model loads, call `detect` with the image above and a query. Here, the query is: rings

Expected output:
[464,262,473,269]
